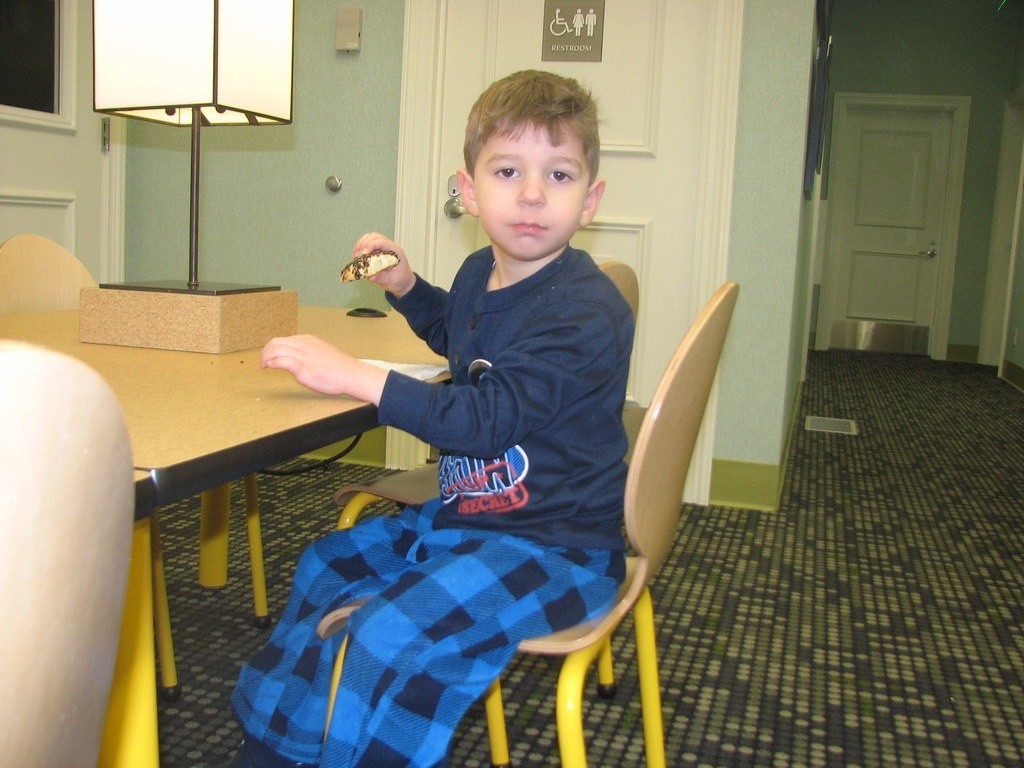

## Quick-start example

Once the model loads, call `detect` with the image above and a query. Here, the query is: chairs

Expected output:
[346,258,737,765]
[0,228,264,765]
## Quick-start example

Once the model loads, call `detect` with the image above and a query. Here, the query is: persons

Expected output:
[228,69,630,768]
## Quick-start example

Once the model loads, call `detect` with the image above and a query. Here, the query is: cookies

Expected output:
[340,250,400,284]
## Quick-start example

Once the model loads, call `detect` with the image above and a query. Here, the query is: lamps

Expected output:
[77,1,296,354]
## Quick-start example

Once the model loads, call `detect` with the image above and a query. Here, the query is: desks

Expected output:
[1,292,474,695]
